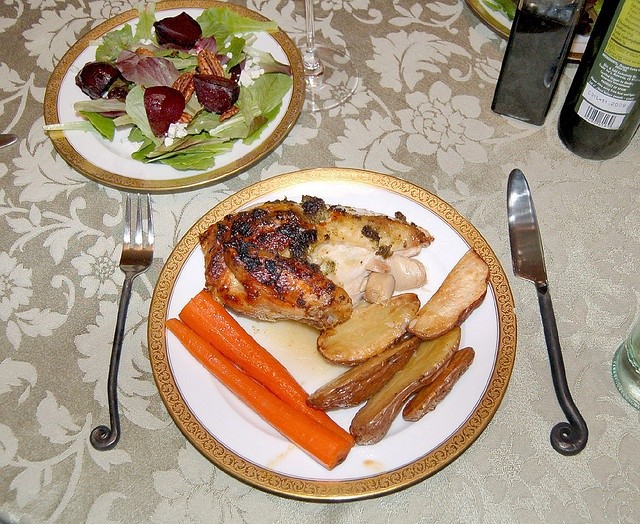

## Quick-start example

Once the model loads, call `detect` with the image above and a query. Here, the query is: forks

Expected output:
[90,194,155,452]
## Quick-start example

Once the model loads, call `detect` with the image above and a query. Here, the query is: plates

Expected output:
[147,166,517,503]
[42,0,305,192]
[466,0,604,61]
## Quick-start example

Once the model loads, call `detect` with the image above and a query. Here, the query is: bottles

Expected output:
[558,0,640,161]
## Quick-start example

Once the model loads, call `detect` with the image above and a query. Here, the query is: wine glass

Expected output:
[297,1,358,111]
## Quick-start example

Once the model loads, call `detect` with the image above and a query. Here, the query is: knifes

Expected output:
[507,168,588,456]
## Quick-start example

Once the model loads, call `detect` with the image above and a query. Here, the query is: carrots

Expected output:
[178,288,356,453]
[163,318,351,470]
